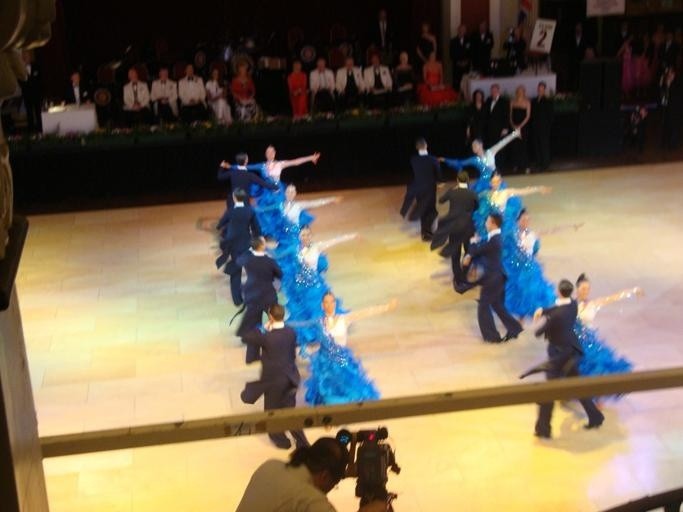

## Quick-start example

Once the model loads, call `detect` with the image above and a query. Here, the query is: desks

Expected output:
[36,101,101,138]
[460,67,560,105]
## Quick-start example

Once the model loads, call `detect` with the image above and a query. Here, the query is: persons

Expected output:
[59,61,256,124]
[465,83,555,171]
[232,439,389,512]
[567,16,680,150]
[397,126,648,440]
[452,22,529,91]
[215,144,383,451]
[286,13,443,123]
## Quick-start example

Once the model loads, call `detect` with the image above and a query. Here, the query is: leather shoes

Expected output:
[423,232,433,240]
